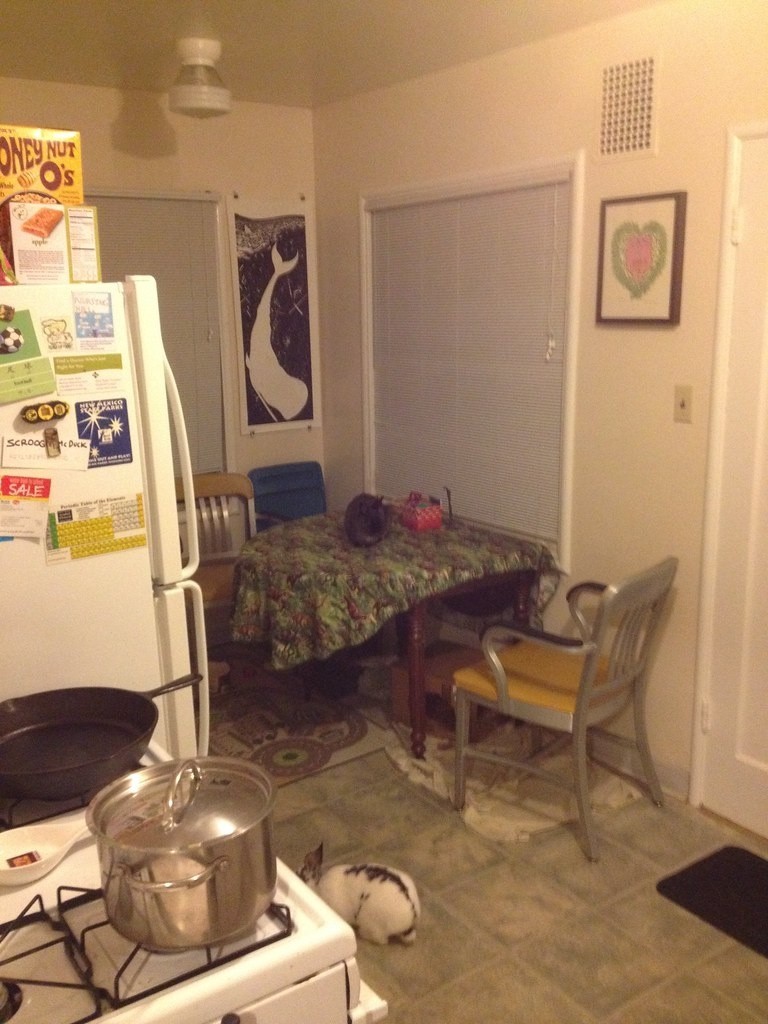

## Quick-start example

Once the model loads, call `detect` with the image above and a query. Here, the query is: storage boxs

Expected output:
[0,124,86,208]
[400,491,443,532]
[0,202,103,283]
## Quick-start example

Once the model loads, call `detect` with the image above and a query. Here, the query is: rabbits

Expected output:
[296,842,420,944]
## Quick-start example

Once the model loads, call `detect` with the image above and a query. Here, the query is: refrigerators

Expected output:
[0,274,209,758]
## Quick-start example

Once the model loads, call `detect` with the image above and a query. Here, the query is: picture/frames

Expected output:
[226,194,322,434]
[594,191,689,324]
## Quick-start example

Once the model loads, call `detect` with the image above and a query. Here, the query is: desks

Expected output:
[246,502,551,756]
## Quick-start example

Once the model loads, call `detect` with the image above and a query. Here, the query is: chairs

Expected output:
[175,472,258,614]
[248,462,327,528]
[457,556,682,859]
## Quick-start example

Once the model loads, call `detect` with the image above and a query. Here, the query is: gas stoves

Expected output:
[0,761,292,1024]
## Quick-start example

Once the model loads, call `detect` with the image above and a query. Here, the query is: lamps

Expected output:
[165,39,231,116]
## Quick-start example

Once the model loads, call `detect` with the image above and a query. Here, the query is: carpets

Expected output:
[203,642,392,785]
[657,845,768,960]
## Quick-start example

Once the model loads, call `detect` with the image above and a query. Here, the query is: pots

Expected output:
[85,756,277,954]
[0,673,203,800]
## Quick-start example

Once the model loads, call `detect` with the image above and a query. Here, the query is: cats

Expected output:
[346,493,390,547]
